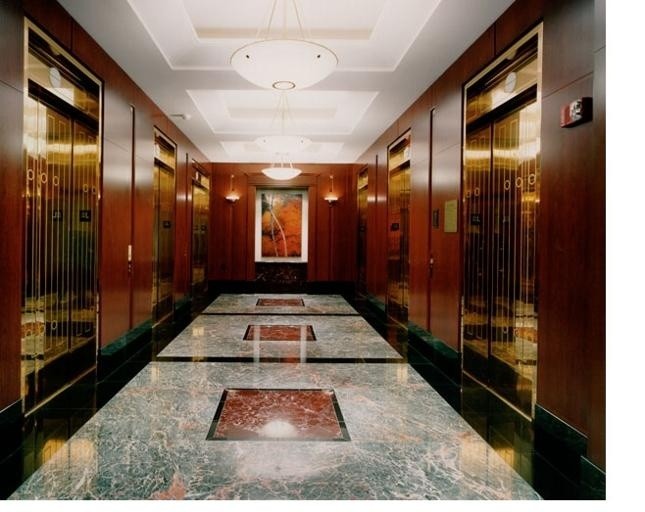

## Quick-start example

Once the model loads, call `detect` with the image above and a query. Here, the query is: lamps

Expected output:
[324,197,338,204]
[225,197,240,202]
[230,0,338,95]
[262,159,302,180]
[254,94,312,159]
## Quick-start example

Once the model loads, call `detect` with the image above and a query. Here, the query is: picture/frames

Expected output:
[254,190,308,264]
